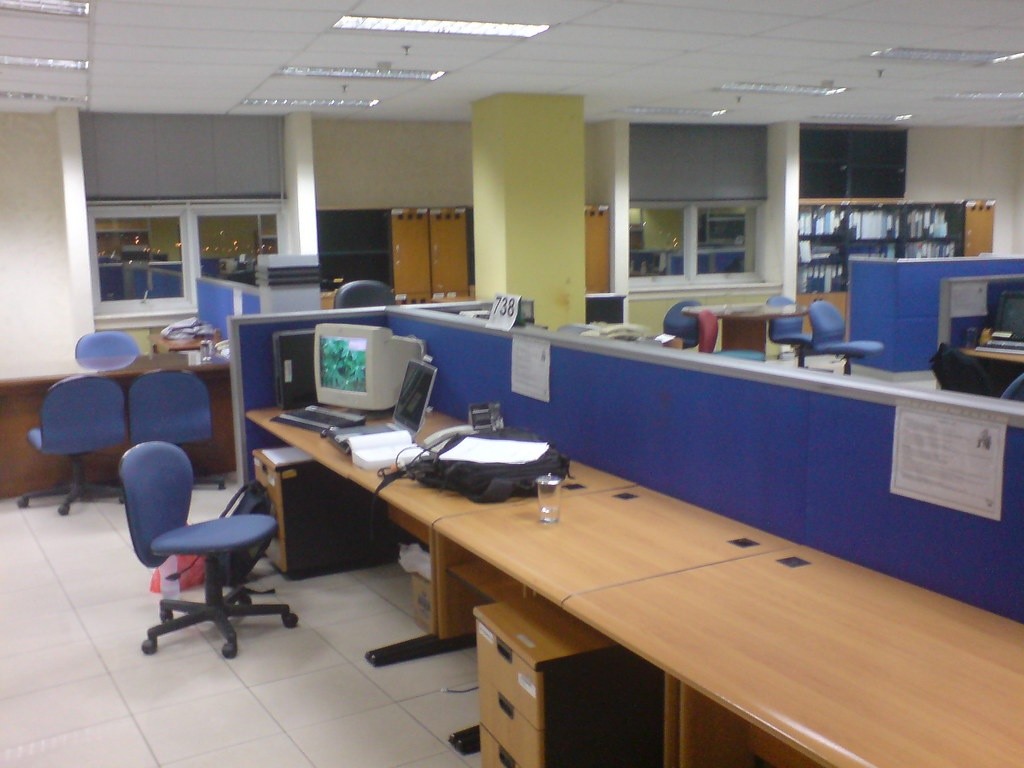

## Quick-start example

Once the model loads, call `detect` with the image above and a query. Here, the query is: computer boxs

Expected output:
[272,330,319,409]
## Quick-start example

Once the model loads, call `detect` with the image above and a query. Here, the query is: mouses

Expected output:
[320,426,339,438]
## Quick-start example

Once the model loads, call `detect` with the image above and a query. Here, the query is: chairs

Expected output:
[17,374,126,515]
[662,300,702,350]
[334,279,395,309]
[766,296,834,374]
[808,301,885,374]
[695,309,766,361]
[117,441,298,659]
[1000,374,1024,403]
[127,368,227,490]
[75,331,141,358]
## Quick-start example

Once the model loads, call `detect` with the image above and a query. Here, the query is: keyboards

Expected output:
[269,405,366,432]
[975,340,1024,355]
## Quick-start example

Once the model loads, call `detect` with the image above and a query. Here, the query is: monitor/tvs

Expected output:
[994,291,1024,343]
[313,324,424,415]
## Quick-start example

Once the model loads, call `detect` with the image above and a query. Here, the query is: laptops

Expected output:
[330,360,438,450]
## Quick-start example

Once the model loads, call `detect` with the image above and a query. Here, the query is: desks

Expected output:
[682,302,809,354]
[959,345,1024,365]
[0,330,238,500]
[244,403,1024,768]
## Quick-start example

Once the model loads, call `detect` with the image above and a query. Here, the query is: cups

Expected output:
[965,327,976,349]
[199,339,212,363]
[536,473,562,522]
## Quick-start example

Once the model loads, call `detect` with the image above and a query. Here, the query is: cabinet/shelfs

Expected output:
[798,198,994,342]
